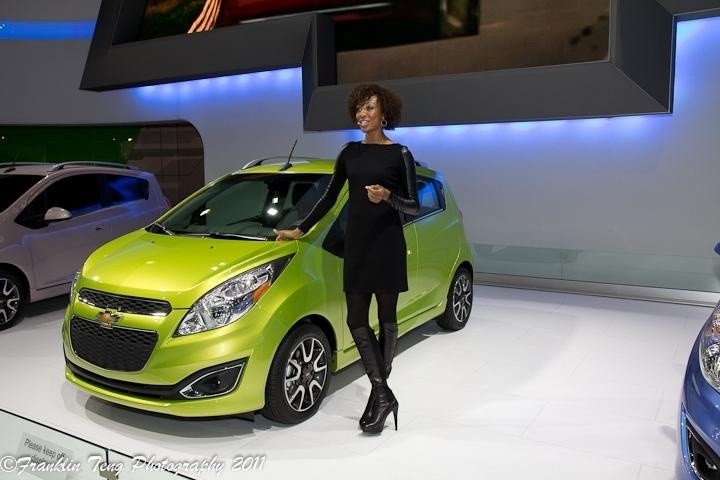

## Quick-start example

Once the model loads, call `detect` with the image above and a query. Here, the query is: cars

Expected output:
[680,240,720,480]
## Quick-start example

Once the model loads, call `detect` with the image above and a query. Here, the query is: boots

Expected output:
[350,322,399,434]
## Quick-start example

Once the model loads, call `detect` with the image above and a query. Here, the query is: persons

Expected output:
[272,83,420,435]
